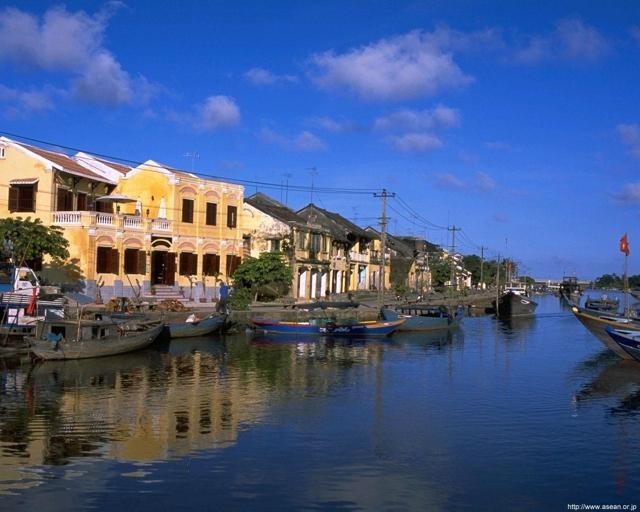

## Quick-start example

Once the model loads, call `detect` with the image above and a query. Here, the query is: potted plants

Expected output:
[211,271,224,302]
[196,271,211,303]
[150,286,157,295]
[94,275,106,305]
[180,269,199,301]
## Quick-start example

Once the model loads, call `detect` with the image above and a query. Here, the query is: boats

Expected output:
[23,317,166,362]
[558,276,640,364]
[246,309,406,336]
[490,277,539,322]
[380,302,464,331]
[95,307,229,338]
[0,283,103,368]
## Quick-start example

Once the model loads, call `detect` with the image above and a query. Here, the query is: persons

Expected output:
[215,279,230,312]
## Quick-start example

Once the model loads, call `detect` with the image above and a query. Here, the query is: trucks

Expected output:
[0,267,41,308]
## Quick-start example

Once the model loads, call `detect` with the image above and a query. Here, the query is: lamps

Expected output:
[116,205,122,214]
[146,208,150,217]
